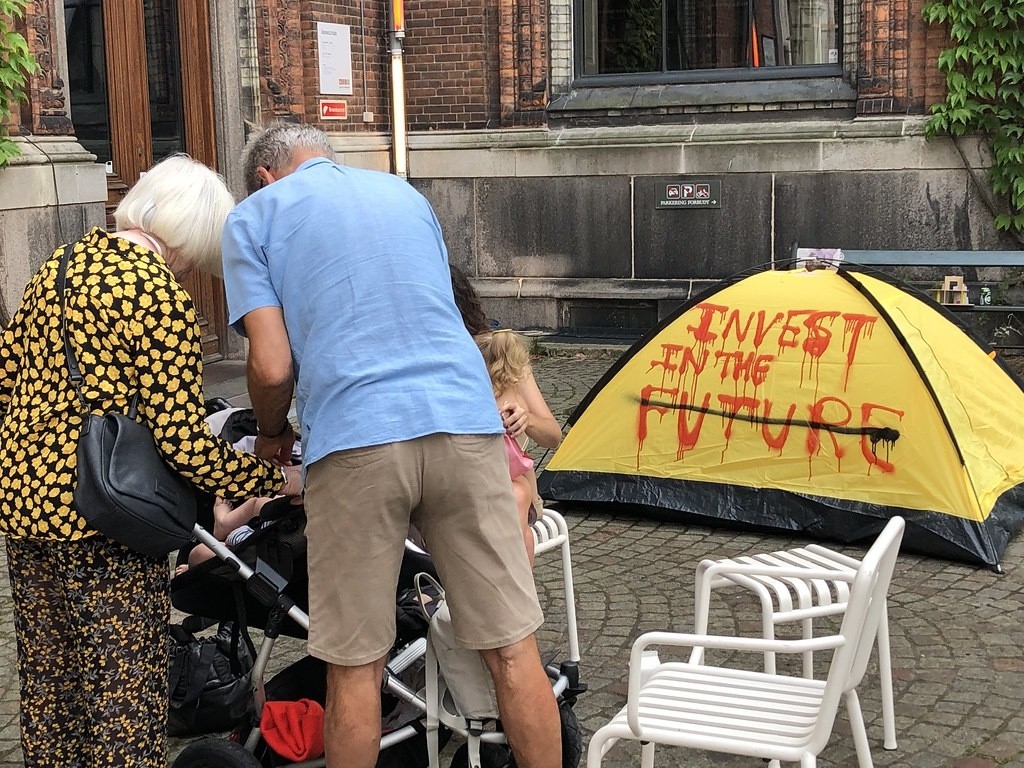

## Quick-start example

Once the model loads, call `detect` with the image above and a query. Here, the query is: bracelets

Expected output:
[281,465,289,484]
[257,417,288,437]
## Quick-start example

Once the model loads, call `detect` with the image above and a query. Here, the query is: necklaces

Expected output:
[139,231,162,256]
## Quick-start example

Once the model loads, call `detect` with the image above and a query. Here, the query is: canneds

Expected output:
[980,288,991,306]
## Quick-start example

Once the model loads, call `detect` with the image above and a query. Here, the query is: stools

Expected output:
[694,544,897,750]
[531,508,580,662]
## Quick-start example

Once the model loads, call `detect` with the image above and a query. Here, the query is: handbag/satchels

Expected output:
[413,571,500,736]
[170,612,259,737]
[74,410,212,561]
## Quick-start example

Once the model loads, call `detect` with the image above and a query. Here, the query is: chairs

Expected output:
[587,515,905,768]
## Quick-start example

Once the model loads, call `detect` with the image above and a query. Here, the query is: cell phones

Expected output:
[502,401,529,452]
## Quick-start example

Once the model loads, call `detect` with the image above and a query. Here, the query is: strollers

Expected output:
[171,396,588,768]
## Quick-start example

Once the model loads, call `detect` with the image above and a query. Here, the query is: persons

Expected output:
[0,152,303,768]
[222,122,561,768]
[174,266,562,576]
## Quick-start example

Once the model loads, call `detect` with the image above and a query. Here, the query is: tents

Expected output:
[535,257,1024,573]
